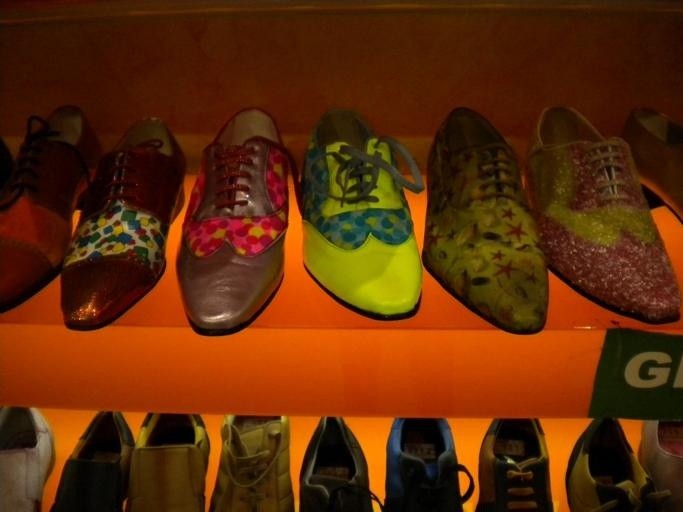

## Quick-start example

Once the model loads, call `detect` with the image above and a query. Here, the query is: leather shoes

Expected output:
[176,104,291,336]
[624,102,681,218]
[1,100,103,313]
[298,104,424,322]
[639,418,682,512]
[525,99,680,324]
[421,108,552,336]
[59,114,187,332]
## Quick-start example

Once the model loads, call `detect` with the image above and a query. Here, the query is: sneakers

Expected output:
[381,419,474,512]
[565,416,667,512]
[125,413,209,512]
[294,418,374,512]
[50,412,137,512]
[1,408,57,512]
[475,420,553,512]
[208,416,294,512]
